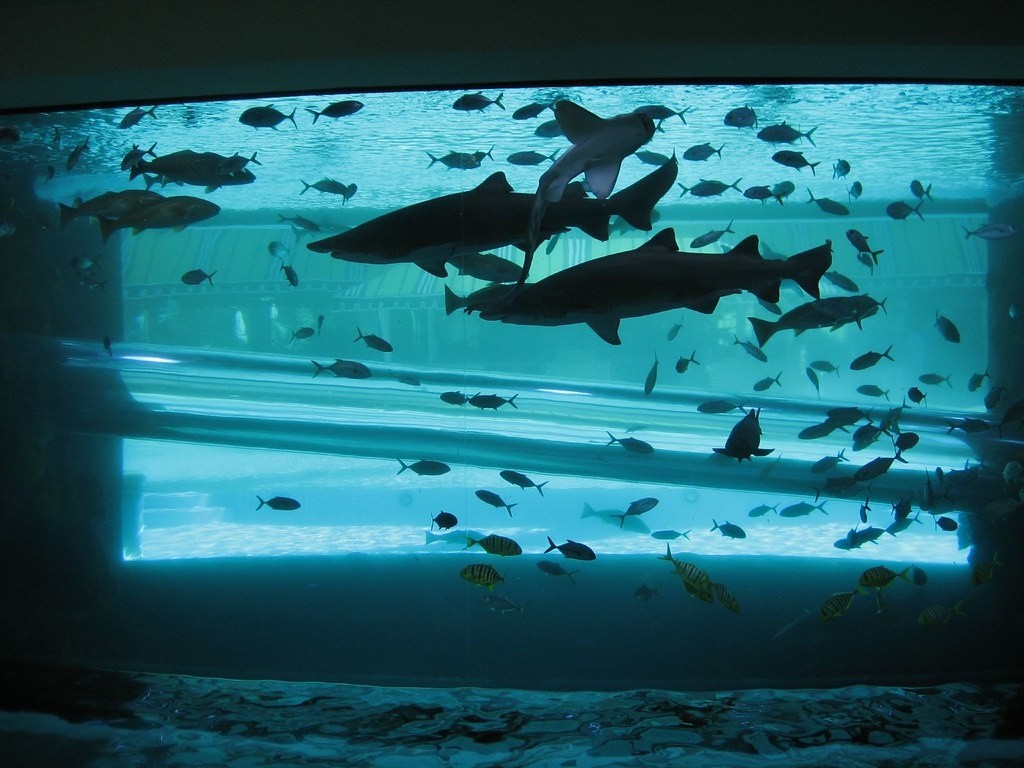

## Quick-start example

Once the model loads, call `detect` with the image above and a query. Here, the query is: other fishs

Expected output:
[0,86,1024,700]
